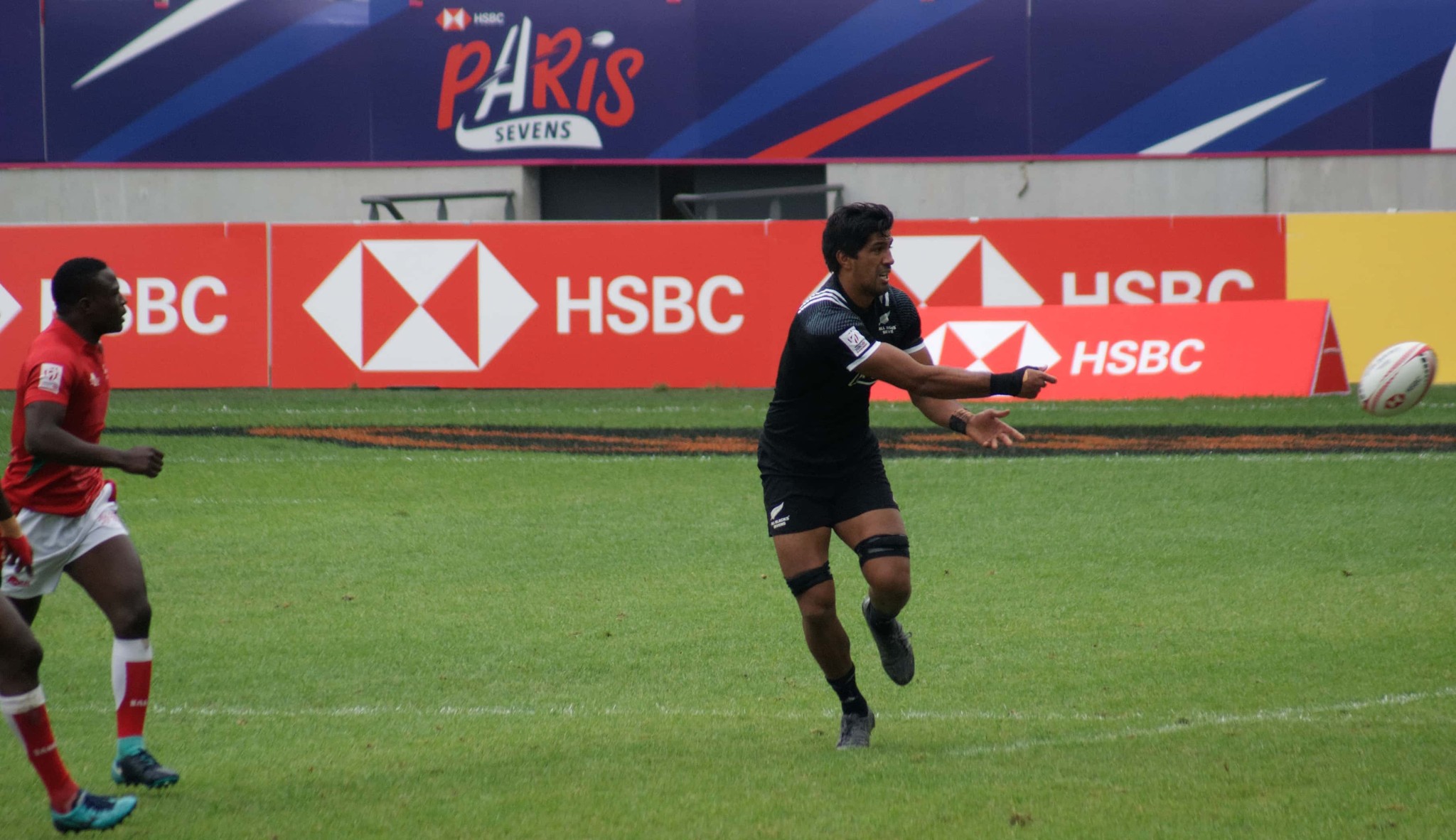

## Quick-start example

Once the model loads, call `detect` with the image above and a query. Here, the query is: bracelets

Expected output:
[948,408,975,436]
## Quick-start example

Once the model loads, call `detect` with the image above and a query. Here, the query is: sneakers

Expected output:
[112,752,181,789]
[837,708,876,749]
[863,594,915,686]
[51,787,139,835]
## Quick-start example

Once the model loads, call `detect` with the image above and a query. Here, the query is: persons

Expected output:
[0,490,138,830]
[0,257,181,790]
[758,201,1056,749]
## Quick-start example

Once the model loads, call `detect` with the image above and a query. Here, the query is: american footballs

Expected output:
[1353,341,1439,415]
